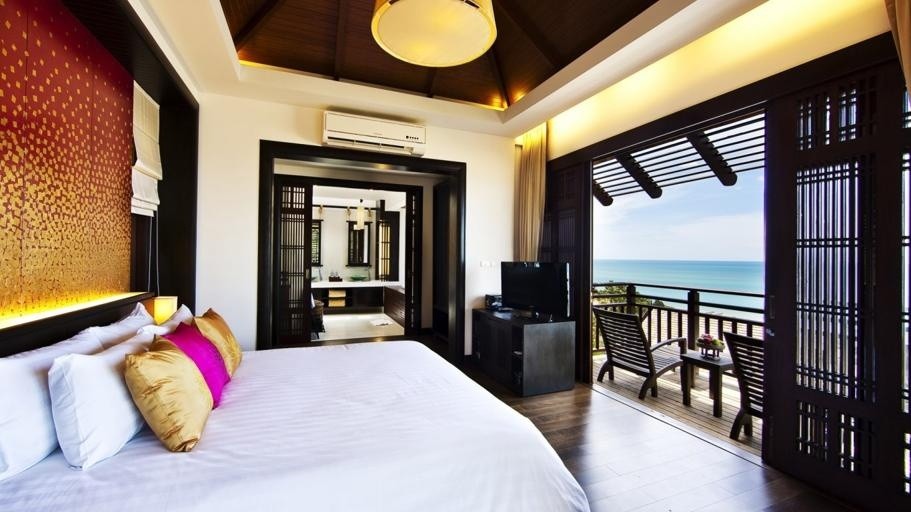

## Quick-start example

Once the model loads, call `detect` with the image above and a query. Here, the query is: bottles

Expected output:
[329,271,343,281]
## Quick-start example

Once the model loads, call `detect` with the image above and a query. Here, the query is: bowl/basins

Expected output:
[351,277,367,280]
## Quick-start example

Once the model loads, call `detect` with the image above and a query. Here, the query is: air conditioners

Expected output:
[321,109,427,158]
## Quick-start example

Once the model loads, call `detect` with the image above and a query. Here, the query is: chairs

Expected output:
[592,306,688,401]
[723,331,765,440]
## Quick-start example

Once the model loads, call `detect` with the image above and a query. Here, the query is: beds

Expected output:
[1,292,594,512]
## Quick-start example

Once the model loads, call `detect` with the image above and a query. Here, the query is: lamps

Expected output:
[371,0,499,70]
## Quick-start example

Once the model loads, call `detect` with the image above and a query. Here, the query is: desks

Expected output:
[311,299,326,339]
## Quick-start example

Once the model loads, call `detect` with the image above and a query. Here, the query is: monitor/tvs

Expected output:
[501,260,570,321]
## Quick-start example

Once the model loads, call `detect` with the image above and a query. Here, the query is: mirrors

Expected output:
[345,221,374,267]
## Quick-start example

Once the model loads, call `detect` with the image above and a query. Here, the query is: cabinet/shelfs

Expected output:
[473,308,576,397]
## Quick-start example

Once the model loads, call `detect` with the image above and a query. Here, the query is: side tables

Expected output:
[680,351,736,417]
